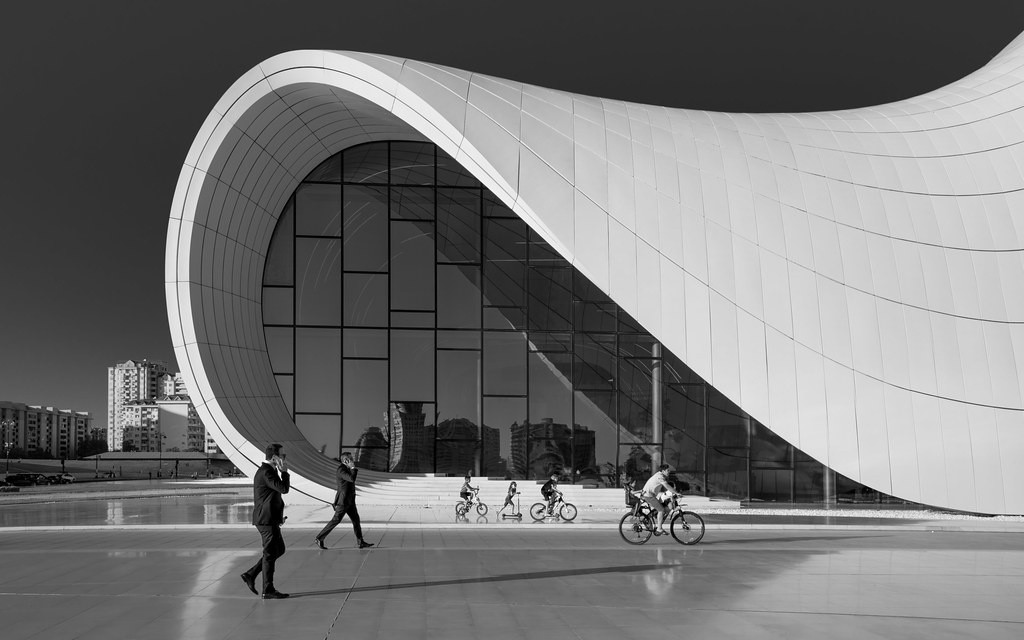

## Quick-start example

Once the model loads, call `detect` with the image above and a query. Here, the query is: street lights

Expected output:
[154,430,168,477]
[2,420,15,475]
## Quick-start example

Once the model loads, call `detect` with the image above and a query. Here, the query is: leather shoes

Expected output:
[262,590,289,599]
[241,573,258,595]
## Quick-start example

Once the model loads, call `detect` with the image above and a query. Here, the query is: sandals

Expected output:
[654,529,669,535]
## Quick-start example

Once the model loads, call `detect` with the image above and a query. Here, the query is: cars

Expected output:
[13,472,75,485]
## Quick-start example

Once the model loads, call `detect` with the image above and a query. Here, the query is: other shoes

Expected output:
[314,538,328,549]
[358,542,374,549]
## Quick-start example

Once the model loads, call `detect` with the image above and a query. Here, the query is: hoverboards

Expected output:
[501,492,522,517]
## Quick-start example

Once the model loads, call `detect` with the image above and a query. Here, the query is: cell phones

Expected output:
[343,458,349,465]
[272,454,281,465]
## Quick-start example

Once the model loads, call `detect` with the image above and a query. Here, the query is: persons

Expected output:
[460,476,478,512]
[109,466,116,478]
[660,481,676,519]
[624,476,646,517]
[496,481,521,516]
[149,470,231,479]
[241,443,290,599]
[541,474,562,517]
[641,463,682,535]
[314,451,375,550]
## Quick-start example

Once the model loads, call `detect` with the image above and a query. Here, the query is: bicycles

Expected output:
[619,494,706,546]
[455,489,488,515]
[530,493,578,521]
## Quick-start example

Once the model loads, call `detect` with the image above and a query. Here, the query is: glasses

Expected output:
[278,453,286,460]
[346,457,353,461]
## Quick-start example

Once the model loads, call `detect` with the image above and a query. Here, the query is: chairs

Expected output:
[624,484,639,515]
